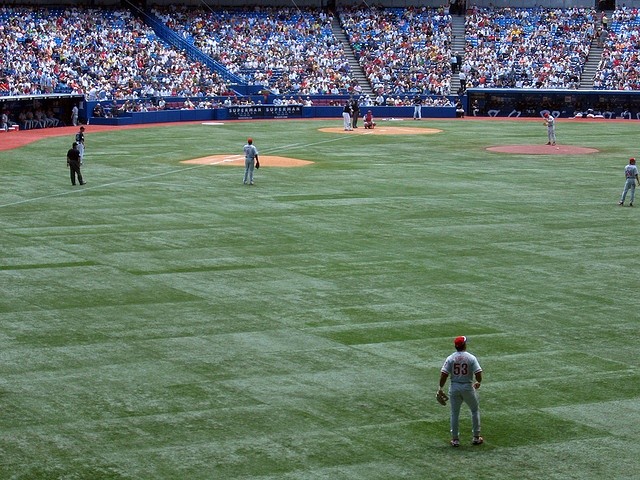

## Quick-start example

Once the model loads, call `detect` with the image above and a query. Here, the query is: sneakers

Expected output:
[450,439,459,446]
[473,437,483,444]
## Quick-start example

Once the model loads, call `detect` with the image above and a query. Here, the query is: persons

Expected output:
[329,0,466,107]
[94,100,234,120]
[439,337,484,447]
[342,100,354,131]
[71,103,79,127]
[67,143,87,186]
[0,4,233,102]
[620,159,640,207]
[234,4,328,116]
[456,1,640,119]
[76,127,86,166]
[543,112,556,146]
[362,109,376,129]
[352,98,359,128]
[243,140,259,184]
[2,108,59,131]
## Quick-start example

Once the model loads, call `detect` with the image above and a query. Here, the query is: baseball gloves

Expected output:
[255,163,259,169]
[543,123,548,126]
[436,390,449,406]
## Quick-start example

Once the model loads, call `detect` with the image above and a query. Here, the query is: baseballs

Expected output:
[557,148,559,150]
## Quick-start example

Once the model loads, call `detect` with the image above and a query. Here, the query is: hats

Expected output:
[454,335,467,347]
[630,158,636,161]
[545,113,549,116]
[80,126,85,129]
[248,138,252,142]
